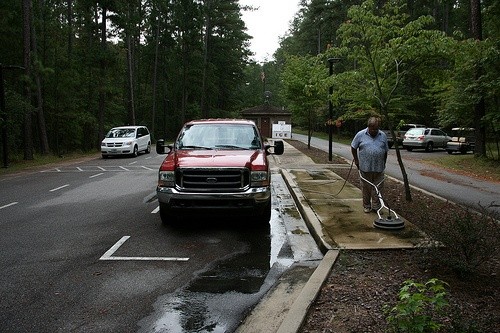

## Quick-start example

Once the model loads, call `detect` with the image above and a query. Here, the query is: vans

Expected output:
[101,126,150,159]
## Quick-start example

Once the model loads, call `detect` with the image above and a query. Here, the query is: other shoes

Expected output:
[363,206,371,213]
[373,209,383,215]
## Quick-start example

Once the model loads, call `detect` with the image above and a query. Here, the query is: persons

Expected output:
[351,117,388,215]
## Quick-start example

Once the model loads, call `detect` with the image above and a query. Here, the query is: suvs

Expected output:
[156,119,285,222]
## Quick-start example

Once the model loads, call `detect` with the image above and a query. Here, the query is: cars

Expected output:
[402,128,452,151]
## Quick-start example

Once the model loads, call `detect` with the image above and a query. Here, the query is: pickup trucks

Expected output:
[387,124,426,148]
